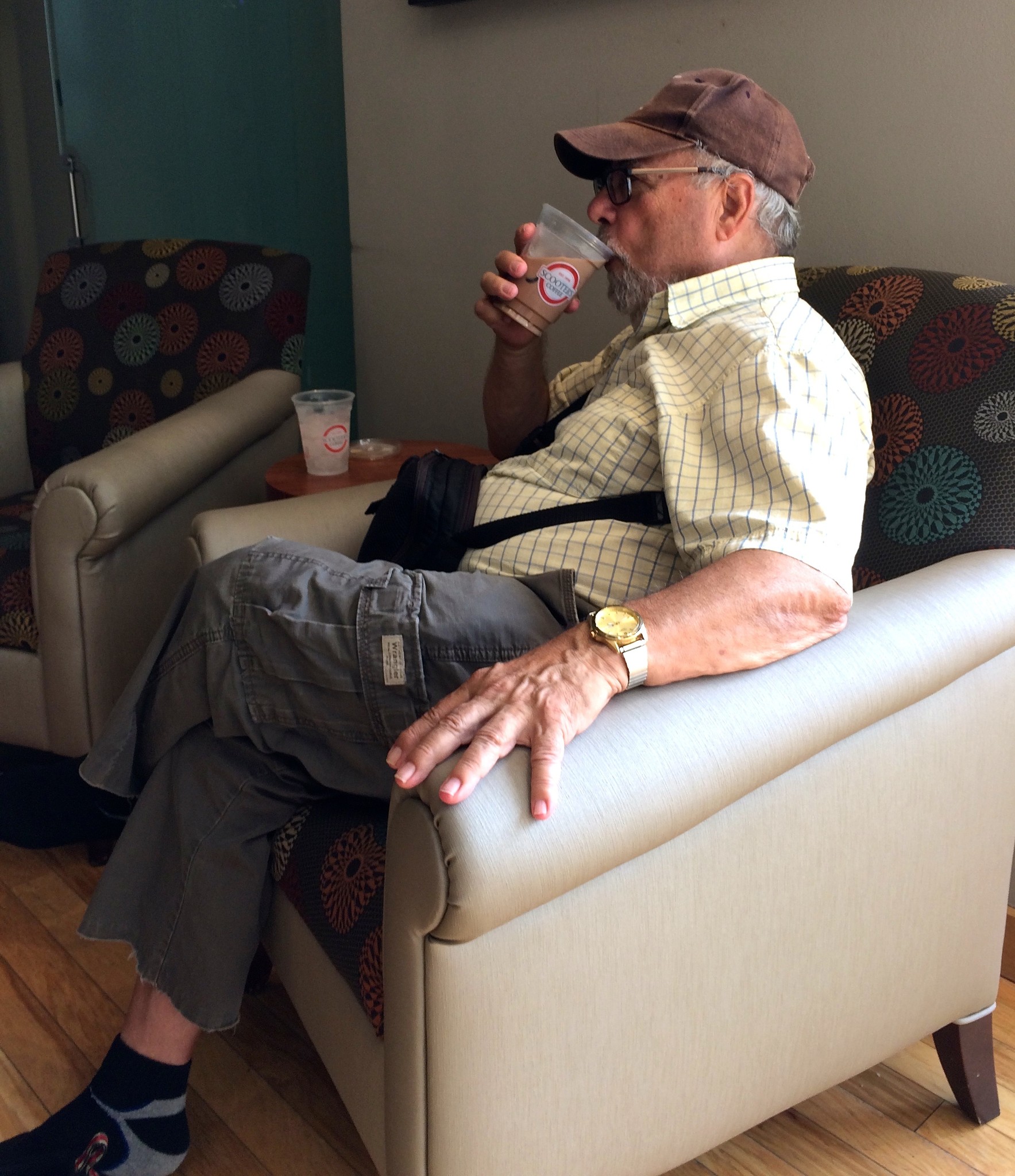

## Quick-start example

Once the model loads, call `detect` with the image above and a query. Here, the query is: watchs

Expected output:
[587,604,649,690]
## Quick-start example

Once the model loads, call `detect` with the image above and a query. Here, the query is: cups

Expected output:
[494,204,616,337]
[291,389,354,476]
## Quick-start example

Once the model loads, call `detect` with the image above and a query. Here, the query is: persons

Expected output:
[0,69,877,1176]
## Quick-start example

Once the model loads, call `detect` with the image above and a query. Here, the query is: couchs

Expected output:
[188,252,1015,1176]
[0,236,316,762]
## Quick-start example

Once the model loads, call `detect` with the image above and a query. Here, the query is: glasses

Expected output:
[593,165,737,206]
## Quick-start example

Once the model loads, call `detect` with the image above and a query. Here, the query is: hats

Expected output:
[553,68,816,207]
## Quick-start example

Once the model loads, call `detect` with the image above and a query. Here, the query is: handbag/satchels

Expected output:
[357,452,489,573]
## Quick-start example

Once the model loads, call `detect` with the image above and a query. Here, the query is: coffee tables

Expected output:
[261,434,495,495]
